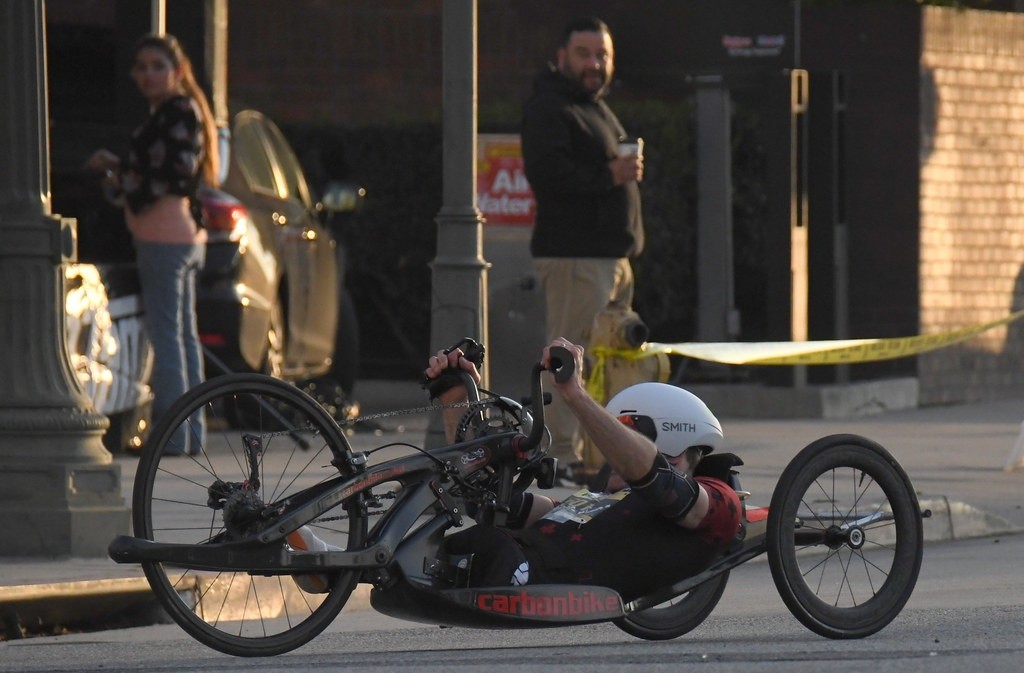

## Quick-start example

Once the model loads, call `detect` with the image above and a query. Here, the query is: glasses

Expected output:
[617,415,640,433]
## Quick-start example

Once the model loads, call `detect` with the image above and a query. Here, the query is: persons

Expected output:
[521,16,642,479]
[289,336,743,604]
[86,31,207,457]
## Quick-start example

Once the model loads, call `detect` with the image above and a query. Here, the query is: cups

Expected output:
[616,135,645,157]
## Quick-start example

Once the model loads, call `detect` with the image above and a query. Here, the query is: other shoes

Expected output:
[127,439,204,457]
[555,462,586,487]
[286,525,346,594]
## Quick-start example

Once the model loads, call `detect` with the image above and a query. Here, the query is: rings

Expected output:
[560,342,565,348]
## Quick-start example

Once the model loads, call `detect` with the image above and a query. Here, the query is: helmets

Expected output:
[603,383,724,458]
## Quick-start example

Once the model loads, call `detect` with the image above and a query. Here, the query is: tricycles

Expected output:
[109,334,934,659]
